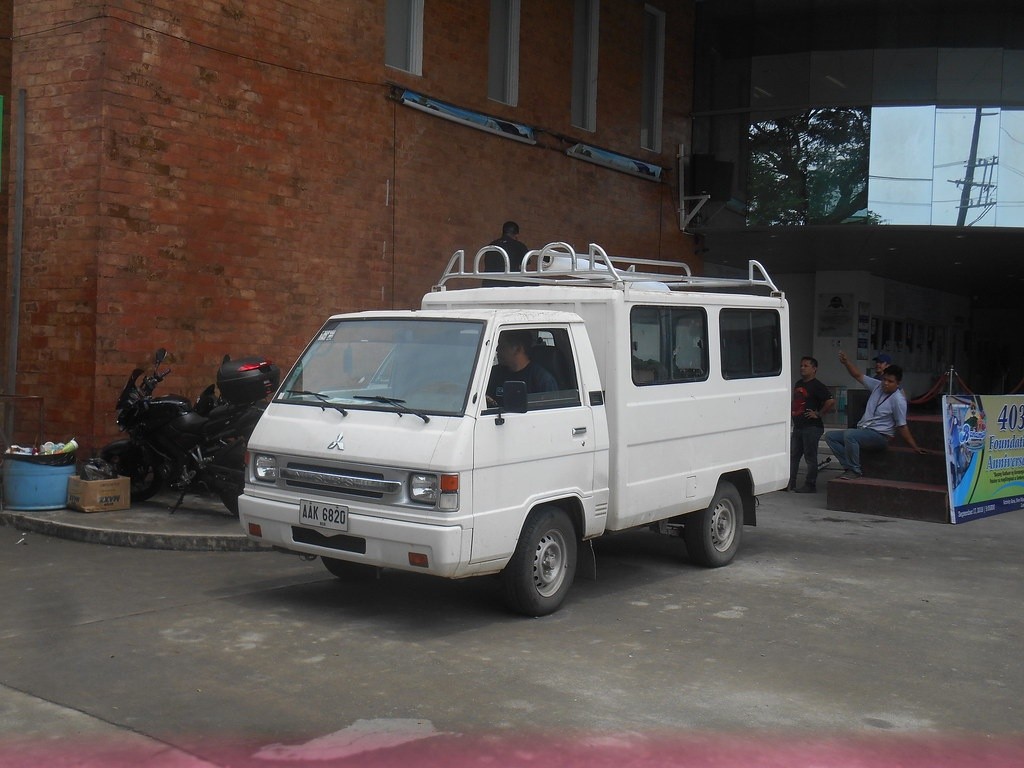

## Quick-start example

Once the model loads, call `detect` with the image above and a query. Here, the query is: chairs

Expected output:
[539,345,567,392]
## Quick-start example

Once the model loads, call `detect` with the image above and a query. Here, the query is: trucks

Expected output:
[236,241,791,618]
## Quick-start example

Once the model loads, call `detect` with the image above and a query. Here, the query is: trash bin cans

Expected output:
[0,437,78,512]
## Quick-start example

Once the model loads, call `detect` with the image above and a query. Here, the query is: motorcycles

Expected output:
[101,347,281,516]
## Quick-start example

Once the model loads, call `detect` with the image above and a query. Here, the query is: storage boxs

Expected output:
[67,473,131,513]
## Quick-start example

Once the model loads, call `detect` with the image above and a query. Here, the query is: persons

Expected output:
[965,402,981,432]
[947,403,960,489]
[824,351,933,480]
[779,356,836,494]
[486,329,558,406]
[482,221,530,287]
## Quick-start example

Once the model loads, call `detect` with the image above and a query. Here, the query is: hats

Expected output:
[872,354,891,364]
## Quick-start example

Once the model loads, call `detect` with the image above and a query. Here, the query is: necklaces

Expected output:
[873,389,898,417]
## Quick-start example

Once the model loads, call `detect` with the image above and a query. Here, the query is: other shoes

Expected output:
[841,468,860,480]
[796,481,816,493]
